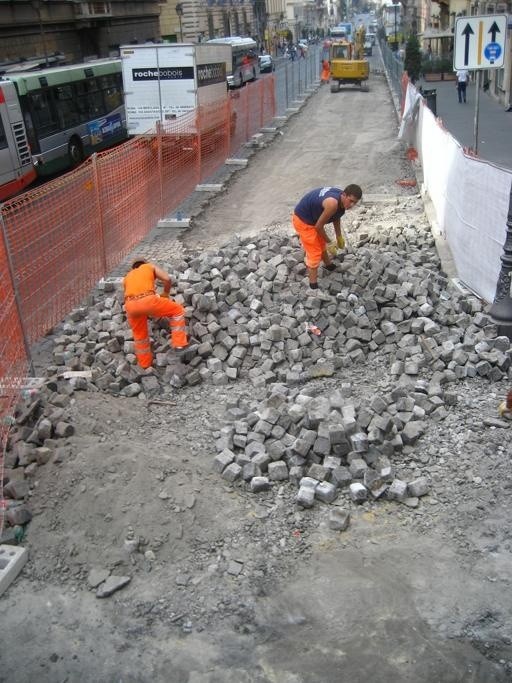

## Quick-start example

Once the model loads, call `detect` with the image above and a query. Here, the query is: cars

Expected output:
[278,7,378,60]
[258,55,276,73]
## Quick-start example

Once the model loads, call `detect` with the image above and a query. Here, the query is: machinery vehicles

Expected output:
[319,22,370,91]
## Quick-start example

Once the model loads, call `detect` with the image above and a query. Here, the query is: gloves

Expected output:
[328,235,344,255]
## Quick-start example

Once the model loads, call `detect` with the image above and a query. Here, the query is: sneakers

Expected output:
[306,287,330,301]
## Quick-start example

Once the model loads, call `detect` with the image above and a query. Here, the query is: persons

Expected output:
[499,388,512,421]
[453,69,471,104]
[123,258,190,369]
[255,12,432,71]
[295,184,362,290]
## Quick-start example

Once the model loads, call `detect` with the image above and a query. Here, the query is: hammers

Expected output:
[335,254,344,263]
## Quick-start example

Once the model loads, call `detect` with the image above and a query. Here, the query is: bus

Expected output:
[204,36,258,88]
[1,70,38,202]
[1,56,147,179]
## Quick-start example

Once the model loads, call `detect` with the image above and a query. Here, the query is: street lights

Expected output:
[392,0,400,81]
[29,0,50,68]
[264,11,271,55]
[175,2,183,42]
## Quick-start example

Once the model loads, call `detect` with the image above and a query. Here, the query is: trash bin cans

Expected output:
[419,86,437,117]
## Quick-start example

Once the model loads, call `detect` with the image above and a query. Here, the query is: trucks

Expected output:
[119,42,237,159]
